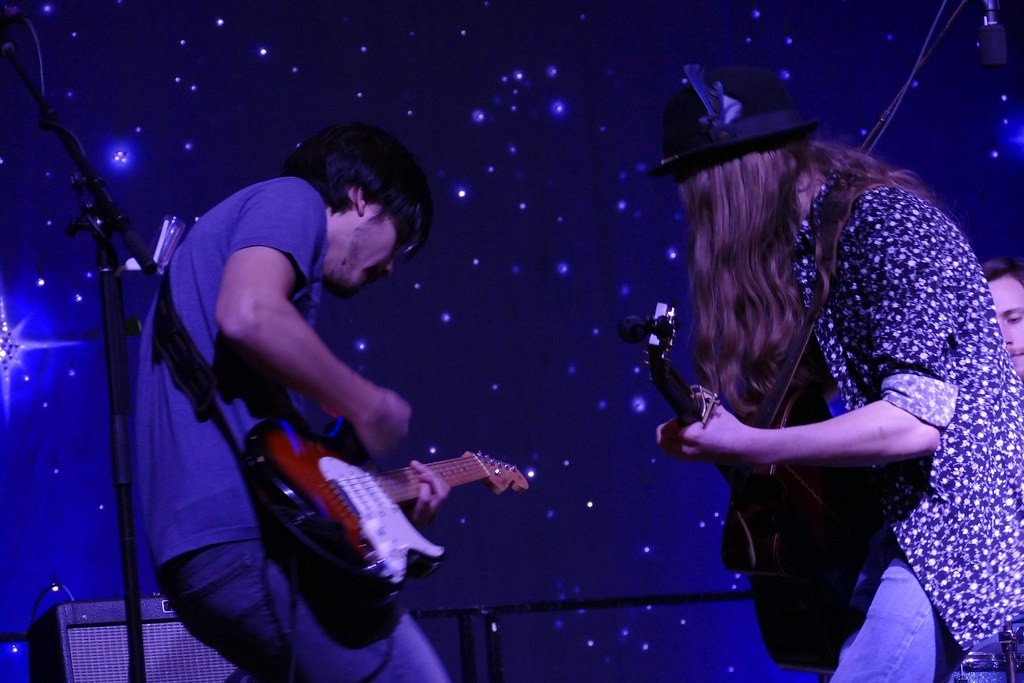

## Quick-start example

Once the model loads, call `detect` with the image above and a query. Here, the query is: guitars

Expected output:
[248,412,532,647]
[618,294,887,672]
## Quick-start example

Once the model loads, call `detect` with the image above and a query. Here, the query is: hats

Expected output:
[648,58,817,177]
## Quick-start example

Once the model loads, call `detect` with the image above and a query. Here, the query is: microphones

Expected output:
[979,0,1007,67]
[0,0,30,26]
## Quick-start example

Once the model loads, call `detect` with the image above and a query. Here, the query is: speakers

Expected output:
[29,598,238,683]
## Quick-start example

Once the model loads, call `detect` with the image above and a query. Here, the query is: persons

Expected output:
[646,64,1024,683]
[134,122,452,683]
[982,254,1024,379]
